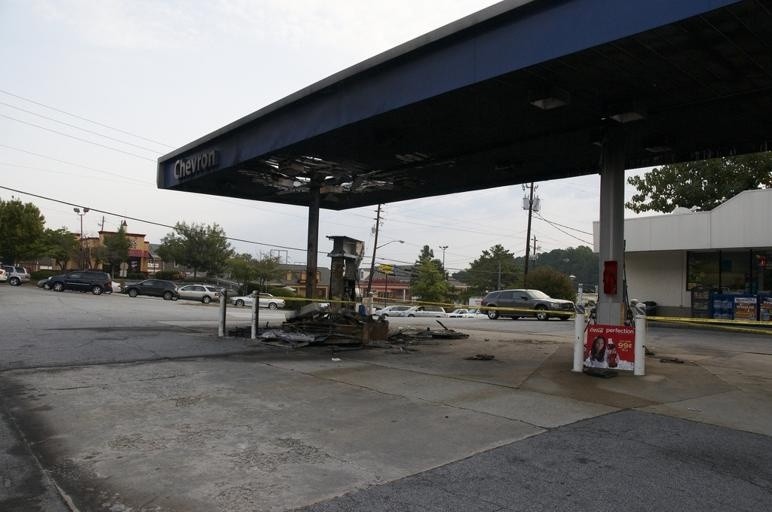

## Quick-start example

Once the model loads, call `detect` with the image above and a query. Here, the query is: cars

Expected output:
[376,304,490,320]
[230,292,286,310]
[0,266,122,295]
[480,289,576,321]
[122,279,180,301]
[177,284,219,304]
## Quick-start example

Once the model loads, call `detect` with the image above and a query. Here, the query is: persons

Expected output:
[584,334,620,369]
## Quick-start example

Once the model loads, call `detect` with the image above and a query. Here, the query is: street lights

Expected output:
[521,195,539,288]
[73,207,89,264]
[367,204,405,299]
